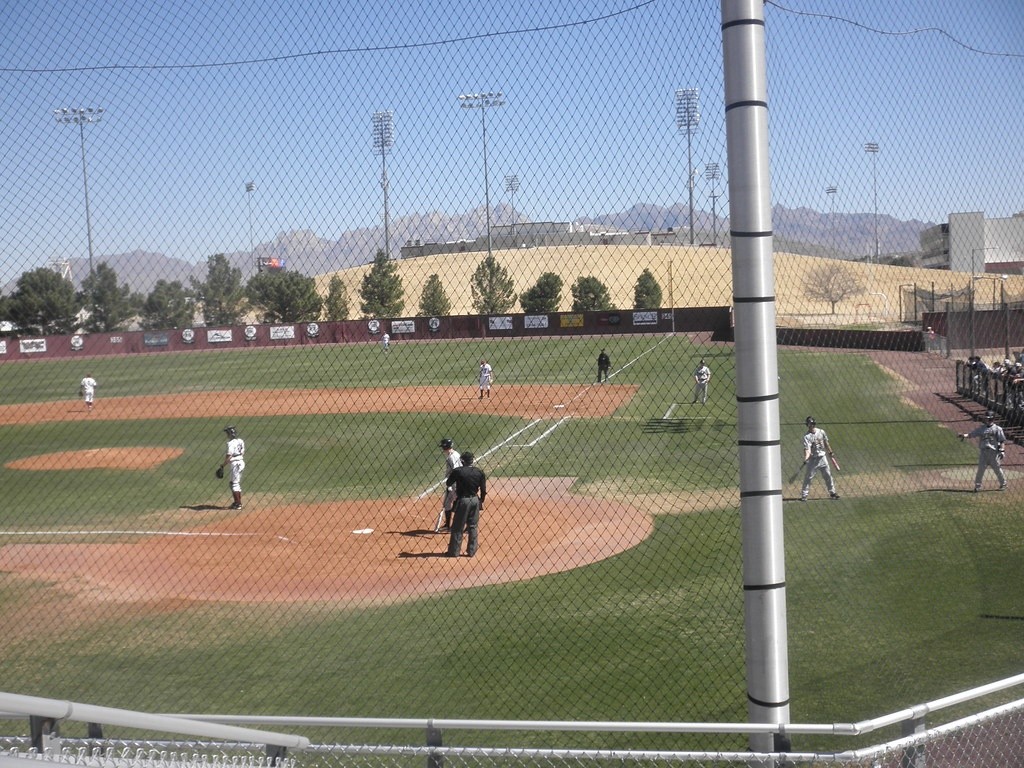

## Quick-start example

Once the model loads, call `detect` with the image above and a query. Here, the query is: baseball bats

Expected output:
[789,453,814,484]
[827,450,840,471]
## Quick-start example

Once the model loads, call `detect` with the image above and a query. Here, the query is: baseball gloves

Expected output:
[79,391,83,396]
[216,465,224,478]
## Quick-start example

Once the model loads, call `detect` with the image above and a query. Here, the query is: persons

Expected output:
[692,360,711,405]
[966,348,1024,412]
[436,438,471,534]
[79,372,97,410]
[596,348,610,383]
[957,410,1008,493]
[800,417,841,501]
[926,327,938,353]
[381,331,390,350]
[221,425,245,510]
[478,360,493,399]
[444,451,487,557]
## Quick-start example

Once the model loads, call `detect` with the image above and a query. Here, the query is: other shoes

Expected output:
[801,497,807,501]
[438,523,469,533]
[998,484,1008,490]
[831,493,840,499]
[228,503,242,509]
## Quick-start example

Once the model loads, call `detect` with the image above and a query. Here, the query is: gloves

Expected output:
[956,433,965,442]
[830,452,834,457]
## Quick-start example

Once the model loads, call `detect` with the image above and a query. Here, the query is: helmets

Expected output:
[700,359,705,364]
[439,439,452,447]
[806,416,816,425]
[984,411,995,418]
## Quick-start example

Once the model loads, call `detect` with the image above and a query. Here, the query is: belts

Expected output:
[460,495,478,497]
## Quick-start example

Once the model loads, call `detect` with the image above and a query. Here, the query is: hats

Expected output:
[460,452,473,463]
